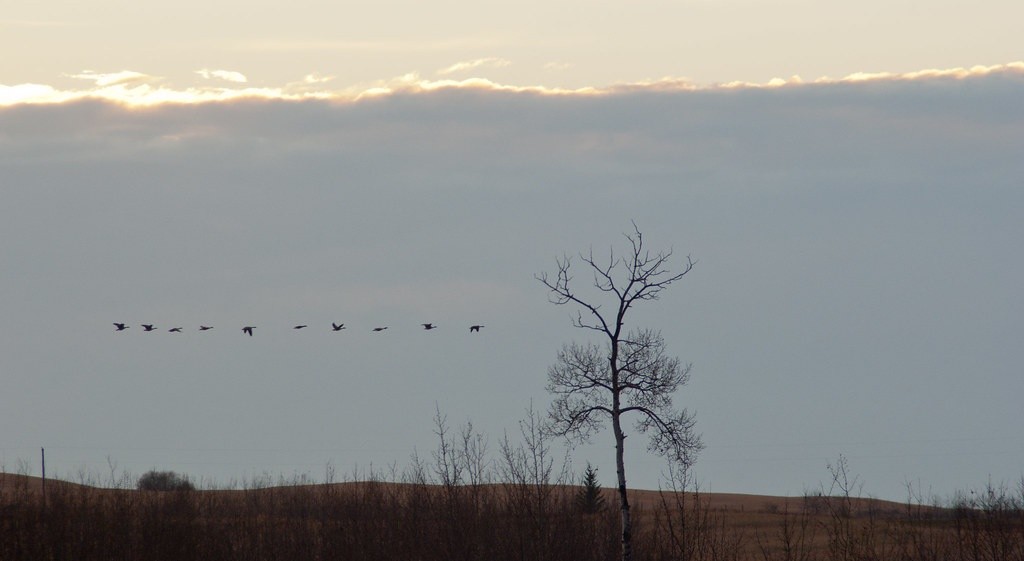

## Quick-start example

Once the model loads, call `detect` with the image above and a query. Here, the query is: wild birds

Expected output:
[469,325,485,333]
[241,326,257,336]
[372,326,389,332]
[168,326,183,333]
[294,325,308,330]
[420,323,438,330]
[112,322,130,331]
[331,321,347,331]
[198,325,214,331]
[141,324,158,332]
